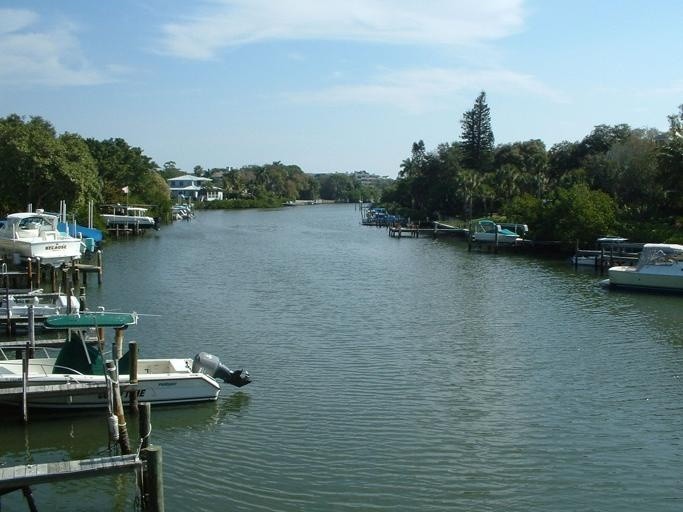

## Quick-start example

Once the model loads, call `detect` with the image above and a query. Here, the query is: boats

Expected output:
[1,203,87,268]
[599,243,683,289]
[473,219,521,244]
[366,207,397,226]
[98,204,162,231]
[1,293,82,325]
[0,311,251,413]
